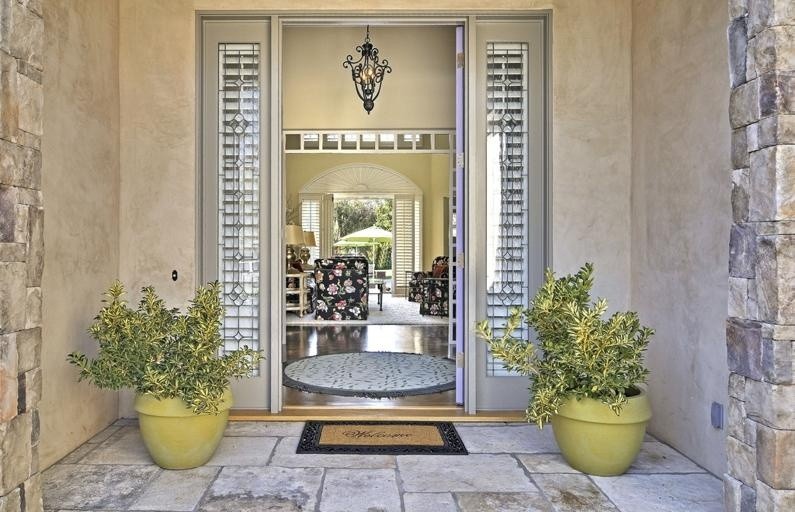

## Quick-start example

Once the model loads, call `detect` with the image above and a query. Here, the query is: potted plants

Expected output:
[472,260,654,475]
[64,278,268,471]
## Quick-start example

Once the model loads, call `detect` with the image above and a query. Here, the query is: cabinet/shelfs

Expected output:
[286,273,312,317]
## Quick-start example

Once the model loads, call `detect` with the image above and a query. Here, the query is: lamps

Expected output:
[285,225,317,273]
[343,25,393,115]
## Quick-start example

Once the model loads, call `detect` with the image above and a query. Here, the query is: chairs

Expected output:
[409,255,456,319]
[314,257,369,320]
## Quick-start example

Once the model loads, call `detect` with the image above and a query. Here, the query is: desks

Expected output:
[370,281,392,310]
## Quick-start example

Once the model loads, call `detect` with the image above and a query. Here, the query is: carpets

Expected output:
[286,294,455,326]
[282,351,456,400]
[295,421,469,454]
